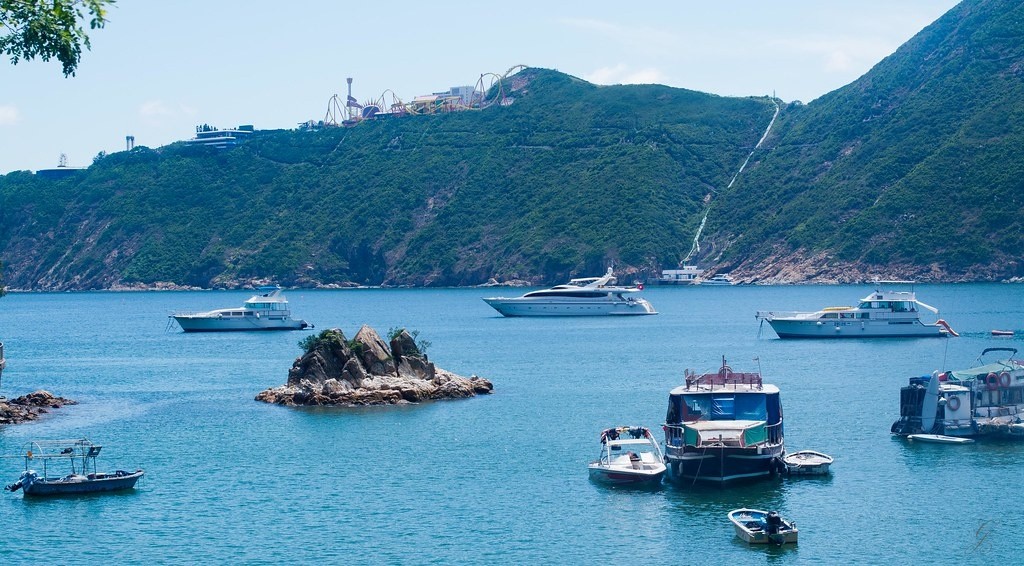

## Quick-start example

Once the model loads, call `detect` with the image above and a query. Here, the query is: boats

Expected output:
[753,257,959,339]
[890,336,1024,440]
[481,266,660,318]
[700,273,736,285]
[782,449,834,476]
[991,329,1014,338]
[167,284,315,332]
[587,425,668,486]
[3,436,146,498]
[726,507,798,548]
[661,355,786,489]
[905,432,976,445]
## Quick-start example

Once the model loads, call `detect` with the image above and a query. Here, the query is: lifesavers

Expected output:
[947,394,961,411]
[999,372,1011,387]
[986,373,999,389]
